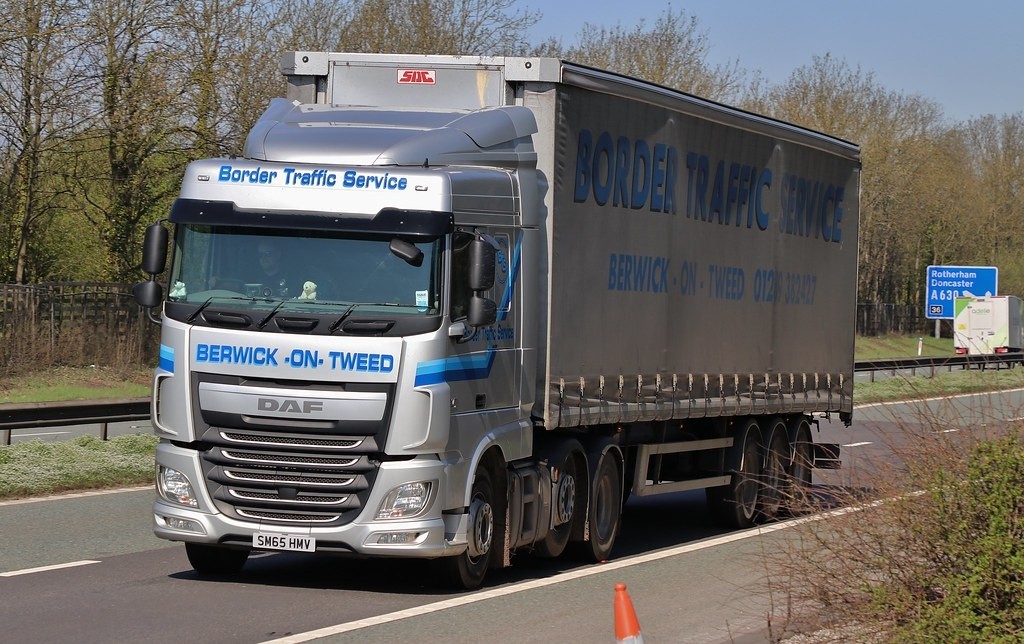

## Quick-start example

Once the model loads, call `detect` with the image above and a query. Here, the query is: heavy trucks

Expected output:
[134,48,863,591]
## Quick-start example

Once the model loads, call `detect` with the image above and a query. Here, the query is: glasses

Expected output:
[256,251,273,257]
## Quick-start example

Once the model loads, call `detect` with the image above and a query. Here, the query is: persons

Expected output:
[246,240,296,299]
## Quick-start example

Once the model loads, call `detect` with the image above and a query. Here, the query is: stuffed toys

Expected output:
[298,281,317,300]
[169,282,186,298]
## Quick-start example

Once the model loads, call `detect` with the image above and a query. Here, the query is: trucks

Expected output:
[954,295,1024,355]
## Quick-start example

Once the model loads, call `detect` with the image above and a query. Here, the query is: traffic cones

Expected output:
[612,582,645,644]
